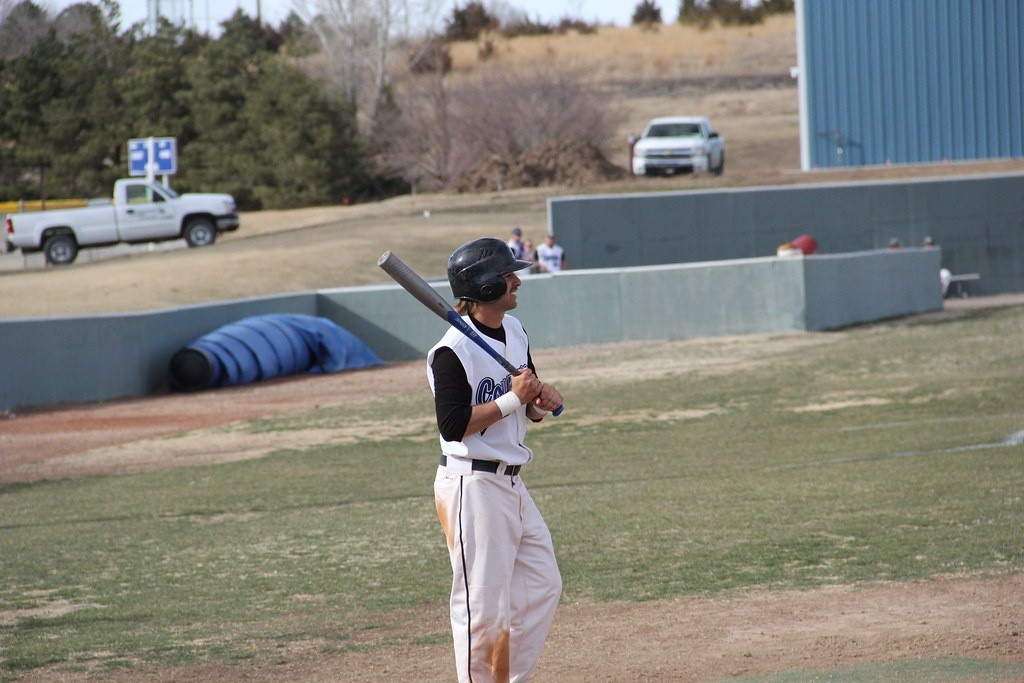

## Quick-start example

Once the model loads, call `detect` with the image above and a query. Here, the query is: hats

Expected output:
[545,231,555,237]
[512,228,523,236]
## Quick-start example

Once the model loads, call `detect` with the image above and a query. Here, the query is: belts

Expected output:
[439,455,521,476]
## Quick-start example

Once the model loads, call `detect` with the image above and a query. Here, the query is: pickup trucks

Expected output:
[5,176,243,266]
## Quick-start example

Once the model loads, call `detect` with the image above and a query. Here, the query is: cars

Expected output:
[632,113,726,181]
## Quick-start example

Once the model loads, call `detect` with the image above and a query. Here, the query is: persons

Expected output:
[923,237,952,296]
[426,238,563,683]
[888,238,900,248]
[508,230,568,272]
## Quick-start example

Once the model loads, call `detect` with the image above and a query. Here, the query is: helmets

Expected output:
[446,237,533,303]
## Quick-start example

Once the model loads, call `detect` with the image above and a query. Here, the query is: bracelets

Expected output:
[495,391,521,416]
[533,405,547,414]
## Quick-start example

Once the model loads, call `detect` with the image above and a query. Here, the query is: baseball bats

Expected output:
[377,250,567,419]
